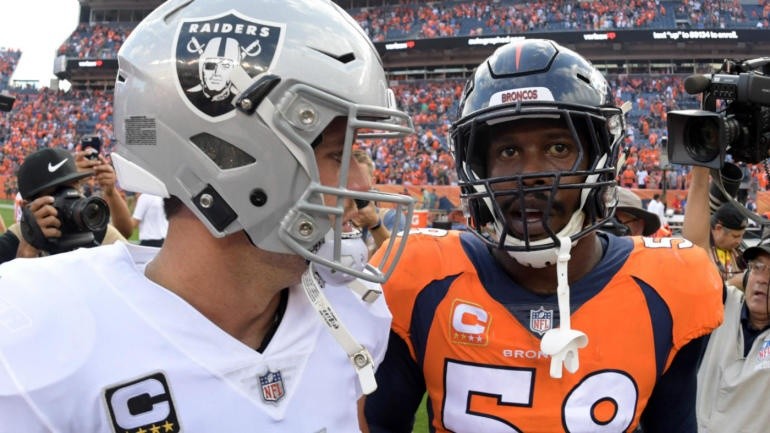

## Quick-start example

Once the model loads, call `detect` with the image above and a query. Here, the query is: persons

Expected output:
[65,144,135,244]
[1,0,397,433]
[1,21,135,198]
[0,147,128,266]
[351,39,724,433]
[703,201,757,291]
[681,161,770,433]
[333,148,410,267]
[127,179,169,248]
[14,188,28,225]
[337,0,770,241]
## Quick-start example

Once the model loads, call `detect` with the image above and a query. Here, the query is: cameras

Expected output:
[41,186,110,242]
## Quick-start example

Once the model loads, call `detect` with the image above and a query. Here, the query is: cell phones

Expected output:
[81,135,101,170]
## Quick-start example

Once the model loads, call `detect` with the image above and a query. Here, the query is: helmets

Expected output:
[107,0,403,144]
[444,37,626,136]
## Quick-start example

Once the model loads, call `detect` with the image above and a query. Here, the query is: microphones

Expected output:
[684,76,710,95]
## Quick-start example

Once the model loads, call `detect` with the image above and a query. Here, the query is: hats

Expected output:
[16,147,96,204]
[614,184,663,236]
[741,240,770,261]
[713,201,750,229]
